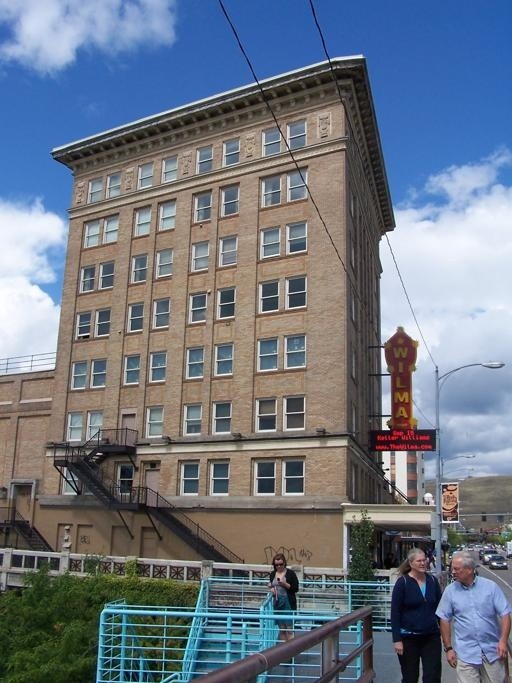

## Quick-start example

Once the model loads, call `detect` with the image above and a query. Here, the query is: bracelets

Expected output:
[444,646,452,653]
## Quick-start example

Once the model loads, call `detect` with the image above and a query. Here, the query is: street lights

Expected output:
[434,361,507,575]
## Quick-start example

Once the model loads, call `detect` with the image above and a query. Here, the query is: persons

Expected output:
[391,548,445,682]
[435,551,512,683]
[267,554,299,664]
[382,545,437,571]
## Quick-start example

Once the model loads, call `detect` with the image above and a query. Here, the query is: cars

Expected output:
[478,548,502,564]
[488,555,508,570]
